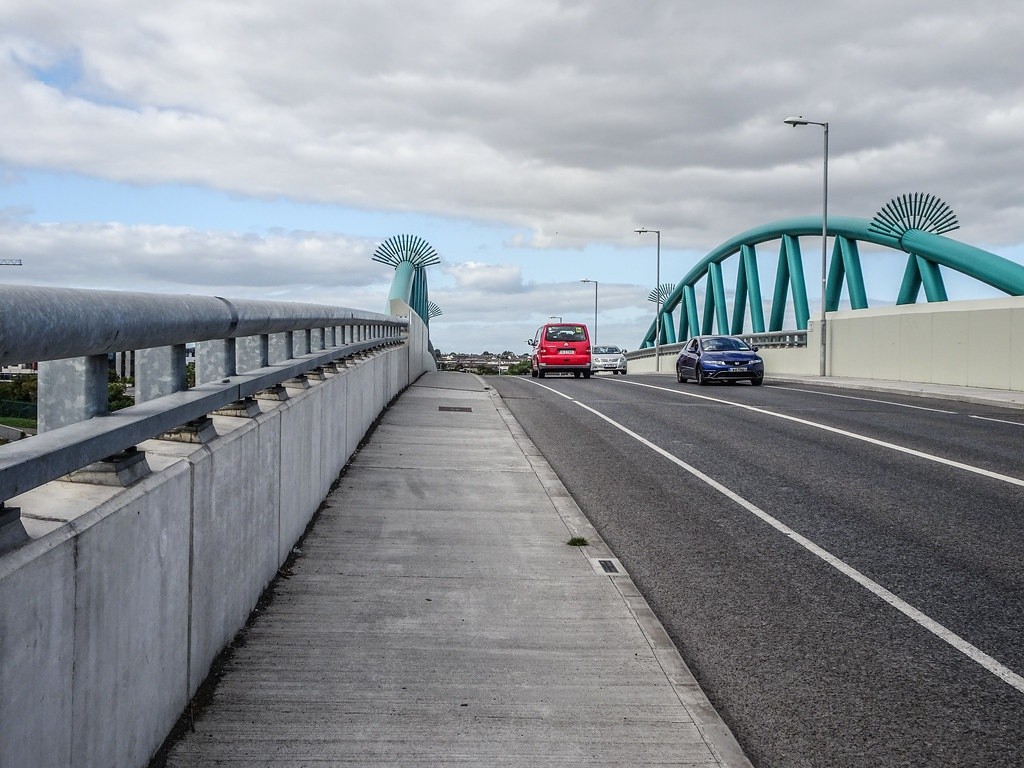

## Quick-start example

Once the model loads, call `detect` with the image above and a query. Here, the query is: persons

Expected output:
[702,340,717,351]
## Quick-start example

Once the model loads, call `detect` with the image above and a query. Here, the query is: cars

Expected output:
[675,335,764,385]
[591,344,628,375]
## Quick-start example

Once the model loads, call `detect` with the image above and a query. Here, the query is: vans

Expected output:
[527,322,591,378]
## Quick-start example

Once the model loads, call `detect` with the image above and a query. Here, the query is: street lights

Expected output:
[783,116,830,376]
[580,278,598,345]
[634,227,661,372]
[549,315,563,323]
